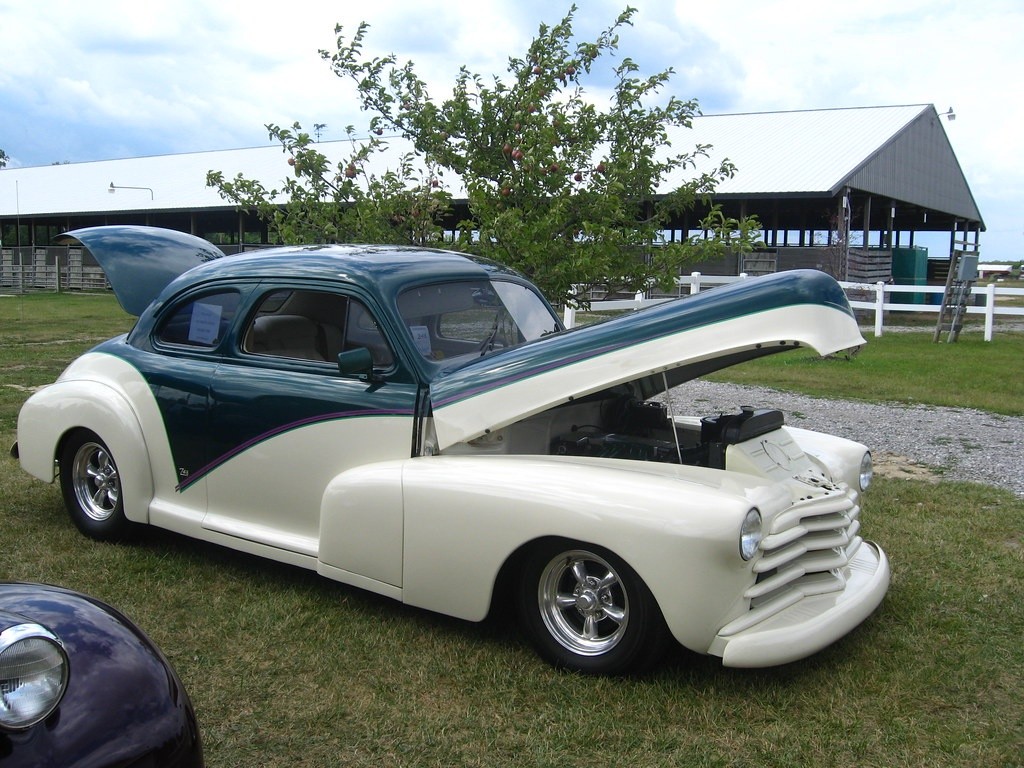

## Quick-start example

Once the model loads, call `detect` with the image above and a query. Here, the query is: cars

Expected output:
[9,226,893,680]
[1,578,207,767]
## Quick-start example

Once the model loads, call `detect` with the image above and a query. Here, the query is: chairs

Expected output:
[254,313,326,361]
